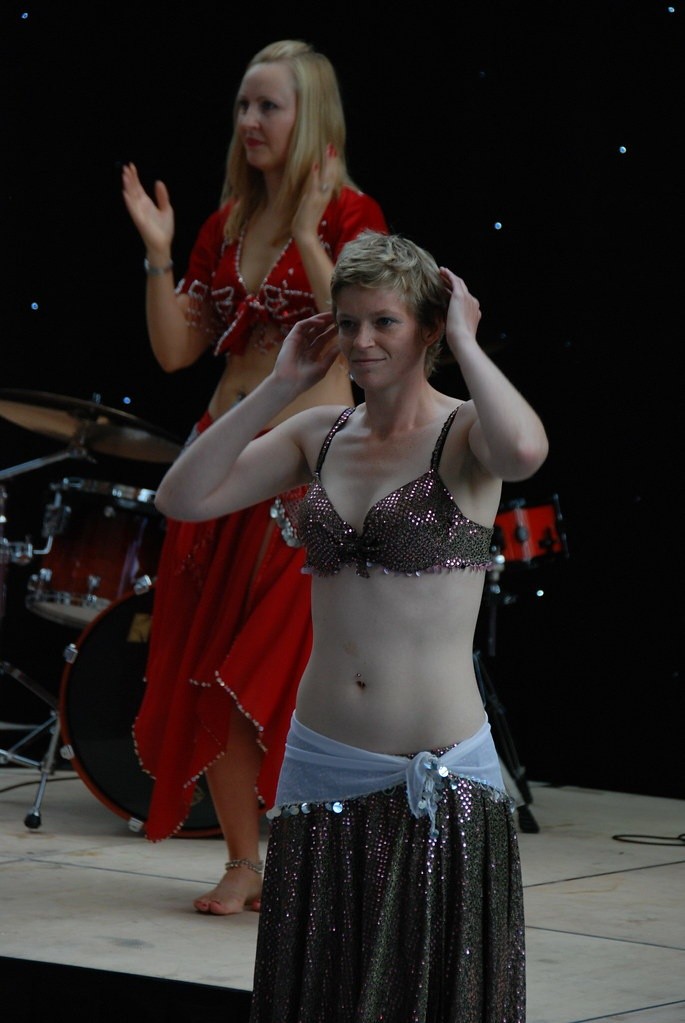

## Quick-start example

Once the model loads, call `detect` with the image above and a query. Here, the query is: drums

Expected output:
[58,576,268,837]
[485,492,569,585]
[25,477,166,630]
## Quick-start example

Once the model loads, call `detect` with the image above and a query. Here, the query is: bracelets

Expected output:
[143,257,176,275]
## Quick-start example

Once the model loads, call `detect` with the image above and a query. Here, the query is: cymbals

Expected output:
[0,388,184,464]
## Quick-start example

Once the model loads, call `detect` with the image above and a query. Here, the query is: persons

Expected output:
[153,229,549,1023]
[121,39,386,914]
